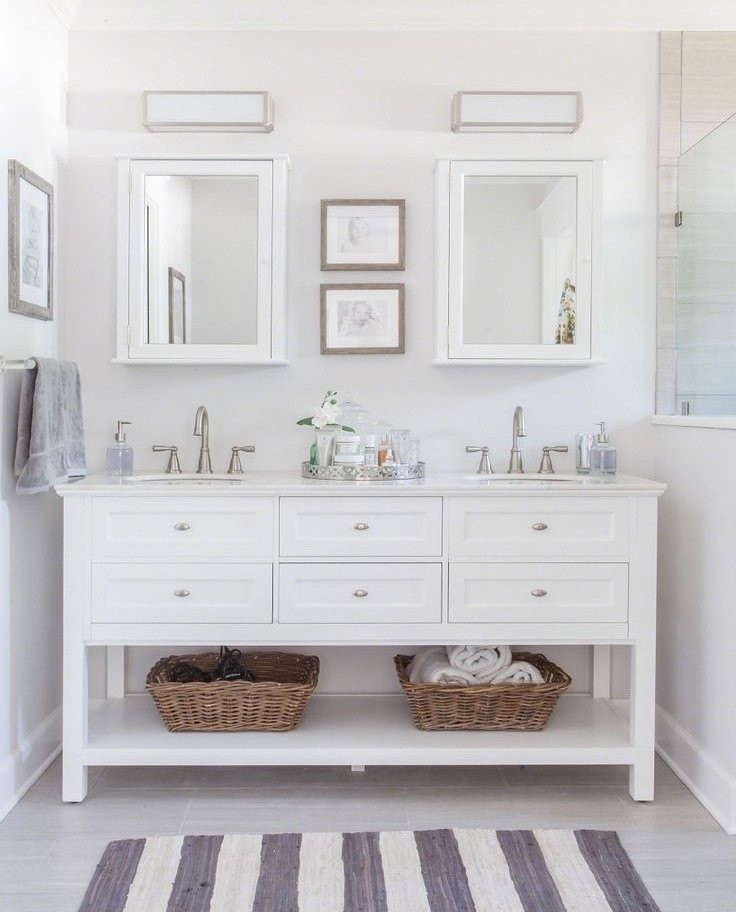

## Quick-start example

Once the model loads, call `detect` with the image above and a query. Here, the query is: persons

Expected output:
[340,301,383,336]
[344,217,386,253]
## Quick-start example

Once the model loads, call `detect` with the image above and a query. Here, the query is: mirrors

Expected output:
[111,152,290,365]
[432,157,608,367]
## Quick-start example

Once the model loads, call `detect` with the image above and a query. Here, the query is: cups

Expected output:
[398,440,419,465]
[579,432,593,466]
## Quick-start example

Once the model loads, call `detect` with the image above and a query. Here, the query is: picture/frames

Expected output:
[320,199,405,271]
[8,159,54,321]
[320,283,405,354]
[168,266,187,344]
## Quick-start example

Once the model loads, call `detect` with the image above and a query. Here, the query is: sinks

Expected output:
[129,472,252,486]
[471,474,582,486]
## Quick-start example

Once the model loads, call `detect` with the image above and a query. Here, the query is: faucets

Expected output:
[193,405,216,474]
[507,406,527,474]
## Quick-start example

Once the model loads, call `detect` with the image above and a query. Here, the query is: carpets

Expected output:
[78,829,661,912]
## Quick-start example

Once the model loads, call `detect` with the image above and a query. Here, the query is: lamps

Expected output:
[449,90,584,135]
[142,89,275,136]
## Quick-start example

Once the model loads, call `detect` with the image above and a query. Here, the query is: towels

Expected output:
[445,645,513,683]
[404,646,481,687]
[493,659,545,685]
[12,357,89,498]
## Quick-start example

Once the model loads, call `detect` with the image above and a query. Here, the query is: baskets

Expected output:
[144,651,319,733]
[393,650,571,730]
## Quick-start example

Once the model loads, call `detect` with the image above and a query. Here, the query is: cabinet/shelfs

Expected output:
[54,473,669,803]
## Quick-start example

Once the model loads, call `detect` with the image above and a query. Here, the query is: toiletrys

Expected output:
[588,421,617,475]
[576,432,591,473]
[315,429,335,476]
[364,435,376,466]
[378,432,389,466]
[106,420,136,476]
[390,429,408,475]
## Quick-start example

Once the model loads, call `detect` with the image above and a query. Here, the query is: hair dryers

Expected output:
[169,647,244,683]
